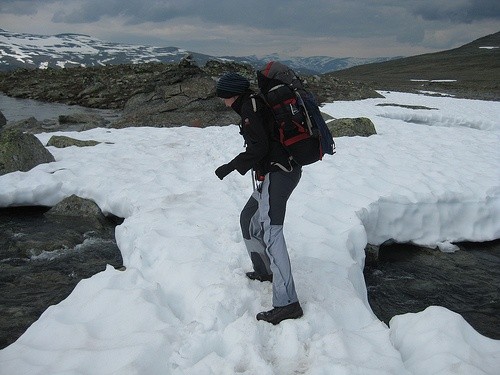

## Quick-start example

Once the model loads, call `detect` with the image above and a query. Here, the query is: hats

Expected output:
[217,73,250,98]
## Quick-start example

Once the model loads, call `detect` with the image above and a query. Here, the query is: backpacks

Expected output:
[254,61,335,166]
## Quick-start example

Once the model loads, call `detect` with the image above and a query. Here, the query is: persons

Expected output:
[215,73,303,325]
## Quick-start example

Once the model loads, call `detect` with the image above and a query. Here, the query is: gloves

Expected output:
[215,163,234,180]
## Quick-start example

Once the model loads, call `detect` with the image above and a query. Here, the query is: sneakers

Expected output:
[256,301,303,324]
[246,272,273,282]
